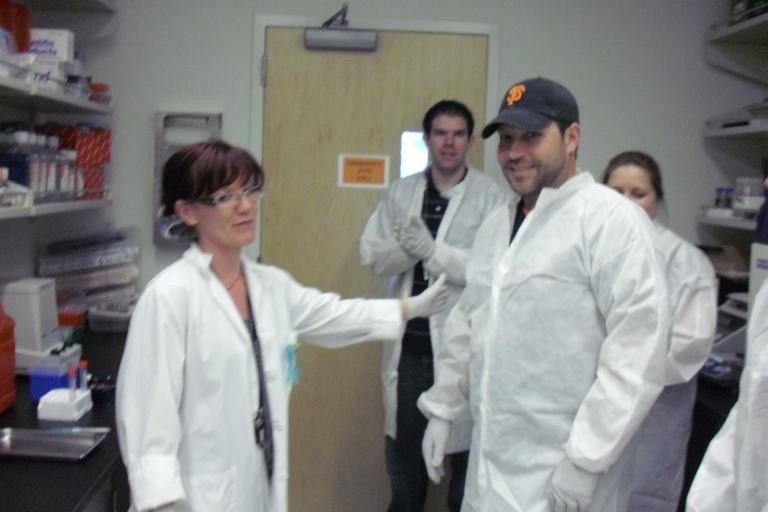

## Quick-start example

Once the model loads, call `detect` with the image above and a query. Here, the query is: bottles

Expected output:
[715,186,733,208]
[67,368,77,402]
[732,176,765,220]
[79,360,88,390]
[65,74,92,98]
[0,130,84,198]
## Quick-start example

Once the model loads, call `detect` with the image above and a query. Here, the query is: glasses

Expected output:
[195,184,262,208]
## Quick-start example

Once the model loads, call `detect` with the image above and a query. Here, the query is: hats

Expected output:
[482,76,580,140]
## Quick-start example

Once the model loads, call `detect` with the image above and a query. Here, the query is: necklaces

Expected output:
[228,272,241,292]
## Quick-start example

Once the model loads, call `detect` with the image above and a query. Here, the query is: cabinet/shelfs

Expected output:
[698,14,768,232]
[0,0,114,224]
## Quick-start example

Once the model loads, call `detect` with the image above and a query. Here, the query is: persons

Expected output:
[114,140,451,512]
[417,77,672,512]
[358,98,509,512]
[601,151,719,512]
[680,276,768,512]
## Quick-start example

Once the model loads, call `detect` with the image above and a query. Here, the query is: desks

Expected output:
[0,328,131,512]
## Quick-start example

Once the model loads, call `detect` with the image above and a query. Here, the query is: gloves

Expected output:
[421,416,449,486]
[546,460,599,512]
[392,211,433,261]
[404,274,450,320]
